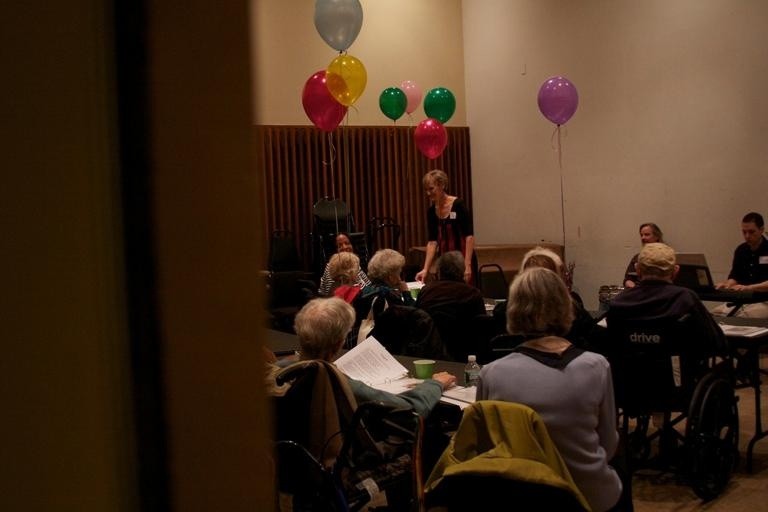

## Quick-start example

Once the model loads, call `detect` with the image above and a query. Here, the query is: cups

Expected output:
[413,358,436,377]
[408,287,420,298]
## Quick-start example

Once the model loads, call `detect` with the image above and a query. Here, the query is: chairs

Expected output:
[476,263,505,293]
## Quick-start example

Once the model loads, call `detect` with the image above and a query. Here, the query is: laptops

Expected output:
[673,264,738,296]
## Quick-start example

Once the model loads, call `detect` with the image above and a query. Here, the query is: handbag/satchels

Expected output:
[356,295,389,346]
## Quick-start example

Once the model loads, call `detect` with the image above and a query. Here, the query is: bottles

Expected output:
[464,354,479,388]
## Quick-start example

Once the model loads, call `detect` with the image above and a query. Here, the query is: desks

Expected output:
[271,291,767,512]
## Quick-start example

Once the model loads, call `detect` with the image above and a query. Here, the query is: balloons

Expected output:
[379,86,407,120]
[399,81,423,114]
[414,119,447,160]
[422,88,455,124]
[538,77,579,126]
[314,0,362,53]
[300,69,350,132]
[326,55,368,105]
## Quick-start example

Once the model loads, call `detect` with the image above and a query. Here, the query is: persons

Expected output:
[607,239,728,466]
[494,247,596,351]
[276,298,456,511]
[712,214,768,367]
[414,170,479,286]
[319,233,415,306]
[418,251,486,358]
[475,269,633,510]
[623,223,663,288]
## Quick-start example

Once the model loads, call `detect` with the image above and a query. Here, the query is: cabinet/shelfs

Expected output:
[409,243,566,290]
